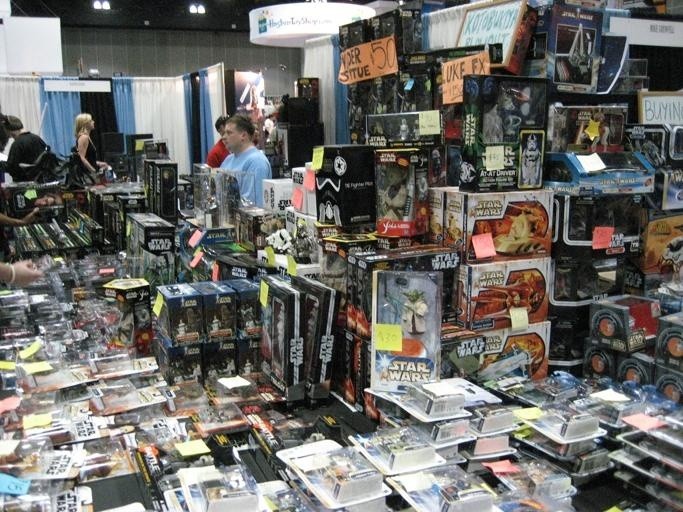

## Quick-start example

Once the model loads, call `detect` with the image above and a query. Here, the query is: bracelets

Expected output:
[6,264,17,285]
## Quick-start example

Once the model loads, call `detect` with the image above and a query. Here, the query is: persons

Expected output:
[0,258,42,287]
[0,207,40,228]
[205,116,233,167]
[3,115,63,185]
[31,193,63,207]
[266,219,293,256]
[214,116,272,210]
[0,112,17,184]
[70,113,117,184]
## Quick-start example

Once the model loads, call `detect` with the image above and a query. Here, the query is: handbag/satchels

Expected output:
[68,155,95,187]
[18,146,56,183]
